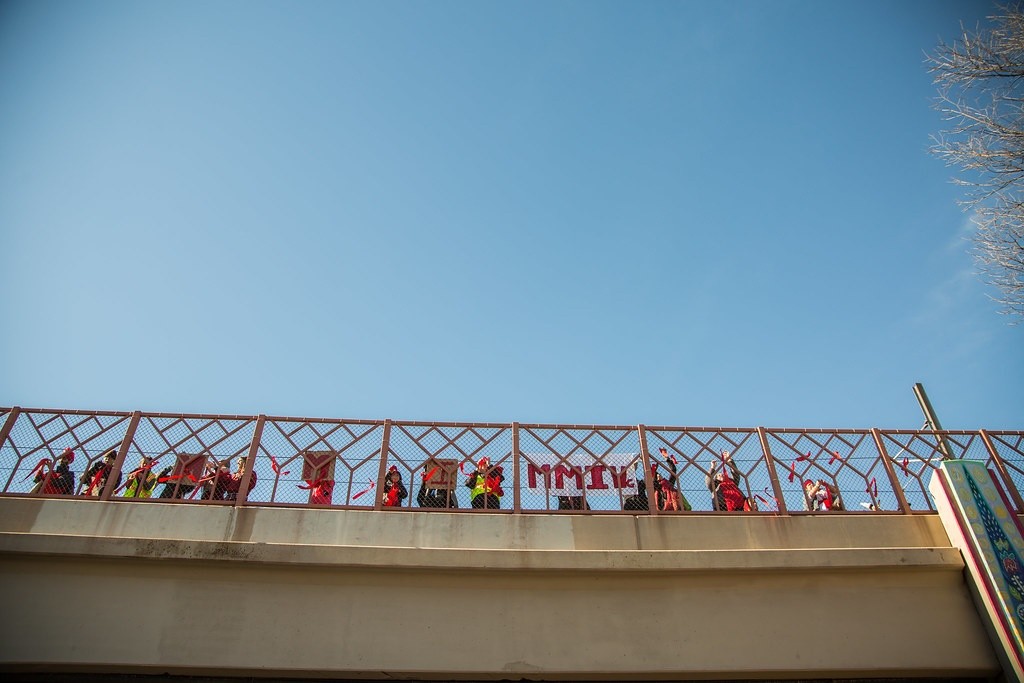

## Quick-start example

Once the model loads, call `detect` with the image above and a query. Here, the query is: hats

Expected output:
[105,450,117,460]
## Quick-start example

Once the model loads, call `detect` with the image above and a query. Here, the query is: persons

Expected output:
[464,457,505,509]
[417,464,459,508]
[223,456,257,501]
[33,448,75,496]
[705,450,748,511]
[623,462,659,511]
[382,465,408,508]
[200,459,232,500]
[157,451,195,499]
[80,450,122,497]
[554,494,591,511]
[649,447,692,511]
[802,478,842,511]
[124,456,157,498]
